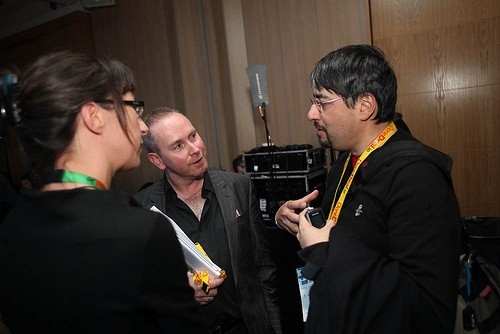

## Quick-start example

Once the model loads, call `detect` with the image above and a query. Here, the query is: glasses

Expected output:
[310,95,357,113]
[92,99,145,118]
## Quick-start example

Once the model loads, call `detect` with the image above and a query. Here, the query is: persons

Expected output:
[0,49,206,334]
[132,107,282,334]
[273,45,464,334]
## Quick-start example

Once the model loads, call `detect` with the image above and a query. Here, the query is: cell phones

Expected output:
[307,207,326,229]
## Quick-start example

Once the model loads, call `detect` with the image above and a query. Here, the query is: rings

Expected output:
[207,297,211,303]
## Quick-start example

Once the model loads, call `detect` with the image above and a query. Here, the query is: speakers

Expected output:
[250,168,331,231]
